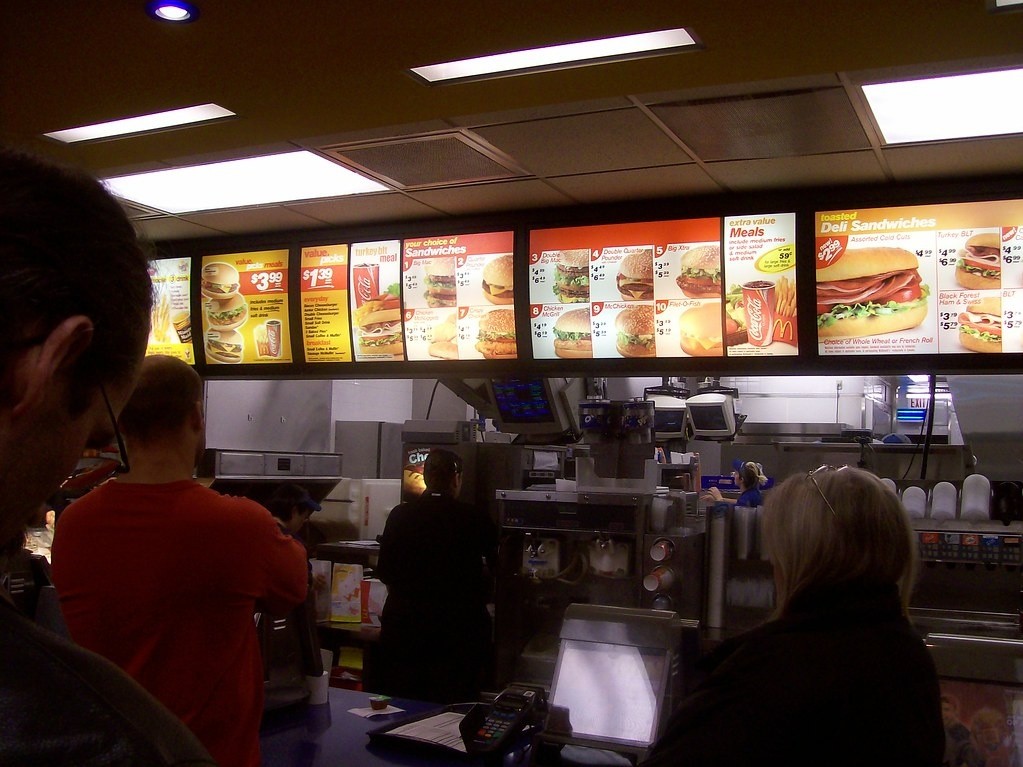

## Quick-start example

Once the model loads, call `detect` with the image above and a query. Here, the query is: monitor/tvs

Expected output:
[482,378,576,443]
[648,393,737,442]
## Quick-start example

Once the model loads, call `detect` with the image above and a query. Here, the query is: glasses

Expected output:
[806,463,848,524]
[50,382,131,504]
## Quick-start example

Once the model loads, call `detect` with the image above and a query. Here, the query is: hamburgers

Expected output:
[957,296,1002,352]
[353,283,403,355]
[481,255,514,305]
[553,250,590,303]
[813,247,929,341]
[615,250,654,301]
[952,233,1000,291]
[676,245,722,298]
[475,309,518,360]
[202,263,247,364]
[679,300,723,355]
[552,306,593,358]
[615,305,656,357]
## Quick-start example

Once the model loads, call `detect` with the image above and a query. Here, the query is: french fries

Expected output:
[770,275,796,317]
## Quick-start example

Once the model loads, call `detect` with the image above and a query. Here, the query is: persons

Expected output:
[376,448,490,701]
[639,469,945,767]
[700,458,767,507]
[0,146,324,767]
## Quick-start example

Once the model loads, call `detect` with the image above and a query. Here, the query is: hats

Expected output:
[733,458,760,489]
[282,482,322,512]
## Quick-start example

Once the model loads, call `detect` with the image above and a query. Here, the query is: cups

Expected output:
[266,320,282,359]
[650,538,673,562]
[305,671,329,705]
[740,280,777,347]
[172,312,193,343]
[643,565,676,593]
[353,264,379,310]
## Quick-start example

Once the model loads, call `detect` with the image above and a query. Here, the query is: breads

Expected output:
[427,313,459,360]
